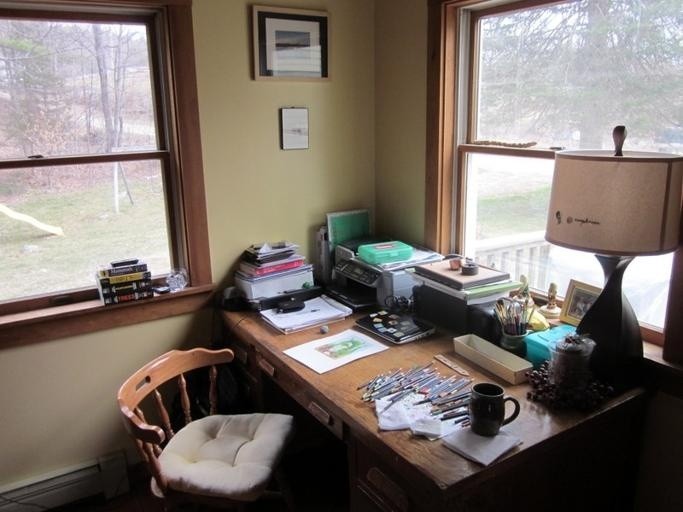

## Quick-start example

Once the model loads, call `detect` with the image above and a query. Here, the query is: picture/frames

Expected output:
[559,280,603,327]
[253,4,332,81]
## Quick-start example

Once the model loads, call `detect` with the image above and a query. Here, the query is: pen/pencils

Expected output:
[357,360,475,427]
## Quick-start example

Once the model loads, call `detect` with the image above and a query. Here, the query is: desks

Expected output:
[218,306,649,512]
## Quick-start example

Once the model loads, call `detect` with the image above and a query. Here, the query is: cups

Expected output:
[469,383,521,437]
[499,326,535,360]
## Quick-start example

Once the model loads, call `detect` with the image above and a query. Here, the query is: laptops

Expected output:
[355,309,438,344]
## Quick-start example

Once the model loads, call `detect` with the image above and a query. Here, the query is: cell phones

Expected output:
[153,285,169,293]
[277,300,305,313]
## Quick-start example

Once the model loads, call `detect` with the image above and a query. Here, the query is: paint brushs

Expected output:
[493,299,536,335]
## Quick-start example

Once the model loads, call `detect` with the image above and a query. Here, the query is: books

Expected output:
[96,258,154,307]
[405,256,521,305]
[234,241,314,284]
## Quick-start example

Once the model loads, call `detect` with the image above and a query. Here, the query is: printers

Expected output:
[328,236,444,318]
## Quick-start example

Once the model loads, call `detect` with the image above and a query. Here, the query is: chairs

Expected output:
[116,347,298,512]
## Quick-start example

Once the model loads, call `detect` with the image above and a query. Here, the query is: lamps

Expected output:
[545,125,683,398]
